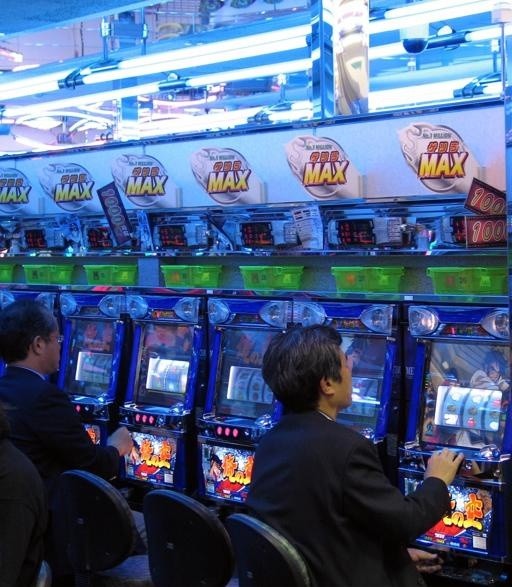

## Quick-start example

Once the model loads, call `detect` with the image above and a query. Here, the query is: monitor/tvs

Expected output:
[336,337,384,432]
[63,317,115,399]
[133,322,195,409]
[213,327,281,420]
[417,339,512,451]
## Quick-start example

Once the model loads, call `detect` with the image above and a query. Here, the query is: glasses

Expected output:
[41,334,65,343]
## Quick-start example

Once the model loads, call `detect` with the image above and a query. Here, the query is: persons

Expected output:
[244,322,464,586]
[1,297,148,557]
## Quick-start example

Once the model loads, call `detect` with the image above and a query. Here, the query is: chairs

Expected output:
[50,470,153,587]
[142,489,239,587]
[226,513,311,587]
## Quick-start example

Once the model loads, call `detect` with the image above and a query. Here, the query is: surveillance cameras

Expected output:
[400,23,429,54]
